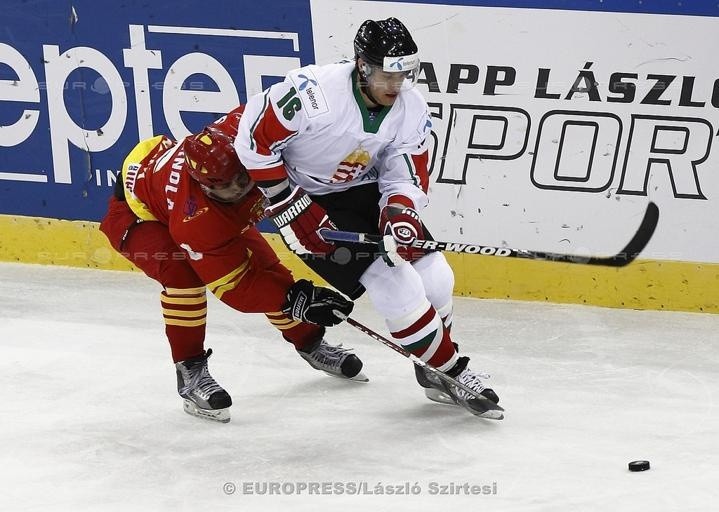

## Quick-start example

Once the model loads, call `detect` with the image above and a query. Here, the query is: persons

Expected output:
[233,16,499,414]
[97,103,364,411]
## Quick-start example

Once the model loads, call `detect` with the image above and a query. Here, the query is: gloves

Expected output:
[281,279,355,327]
[262,184,339,257]
[379,195,427,267]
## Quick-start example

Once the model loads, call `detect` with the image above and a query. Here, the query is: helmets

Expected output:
[183,126,244,190]
[353,16,420,82]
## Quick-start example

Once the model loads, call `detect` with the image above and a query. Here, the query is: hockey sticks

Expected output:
[320,201,659,268]
[332,309,506,419]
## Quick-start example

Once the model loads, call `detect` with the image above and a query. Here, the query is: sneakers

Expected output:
[296,337,363,379]
[414,341,499,412]
[176,347,232,410]
